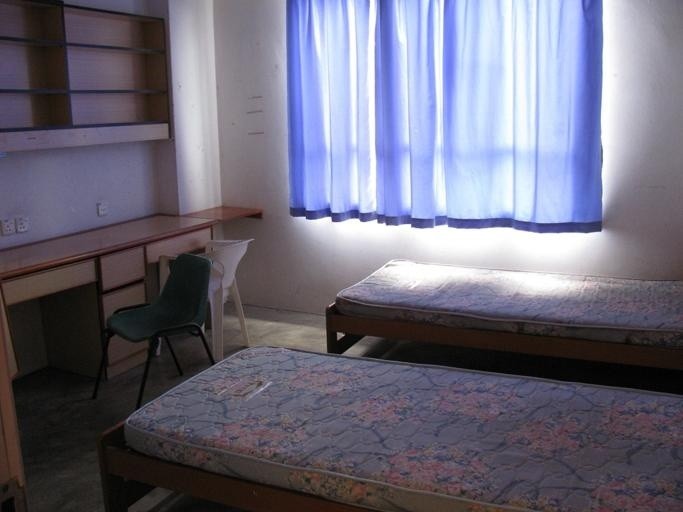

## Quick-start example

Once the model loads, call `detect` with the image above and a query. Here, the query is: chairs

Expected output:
[156,238,255,361]
[93,253,216,411]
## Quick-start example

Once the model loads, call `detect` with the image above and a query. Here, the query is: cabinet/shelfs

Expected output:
[0,0,172,151]
[0,205,263,379]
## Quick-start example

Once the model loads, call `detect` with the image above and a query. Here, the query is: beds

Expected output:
[97,346,683,512]
[326,259,683,370]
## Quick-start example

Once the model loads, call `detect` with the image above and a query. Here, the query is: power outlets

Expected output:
[17,217,28,233]
[2,218,15,235]
